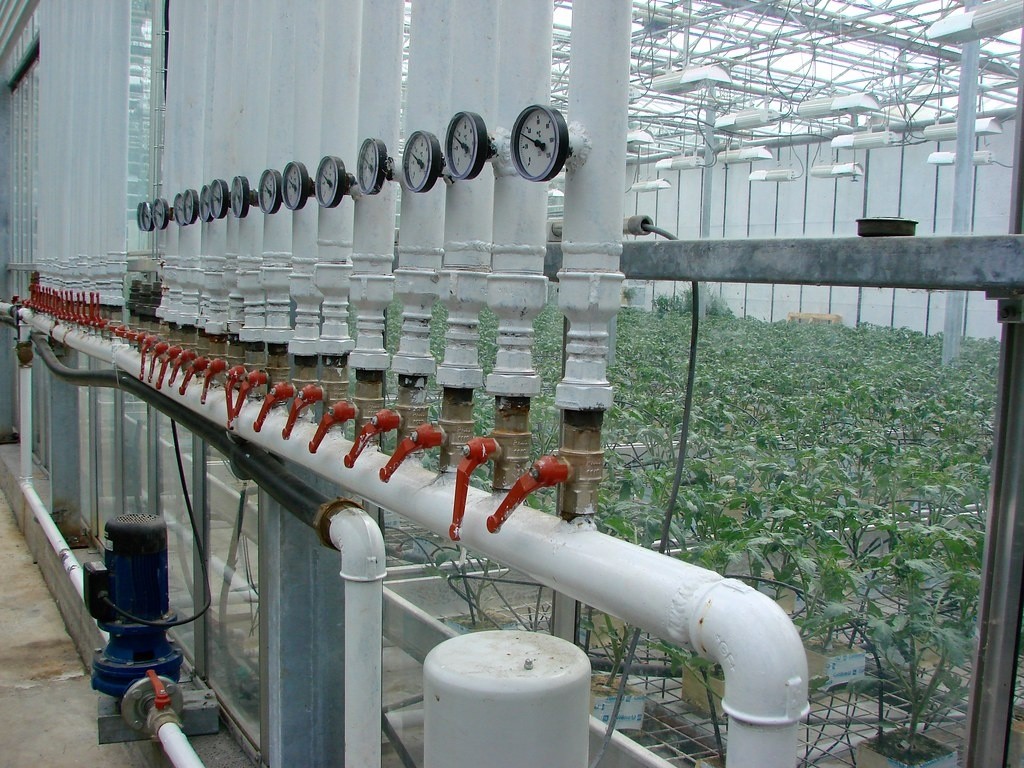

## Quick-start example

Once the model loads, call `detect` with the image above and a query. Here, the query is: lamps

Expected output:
[543,0,1024,217]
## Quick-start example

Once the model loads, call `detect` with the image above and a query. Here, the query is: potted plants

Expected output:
[826,508,977,768]
[751,482,798,615]
[664,527,727,719]
[581,608,645,730]
